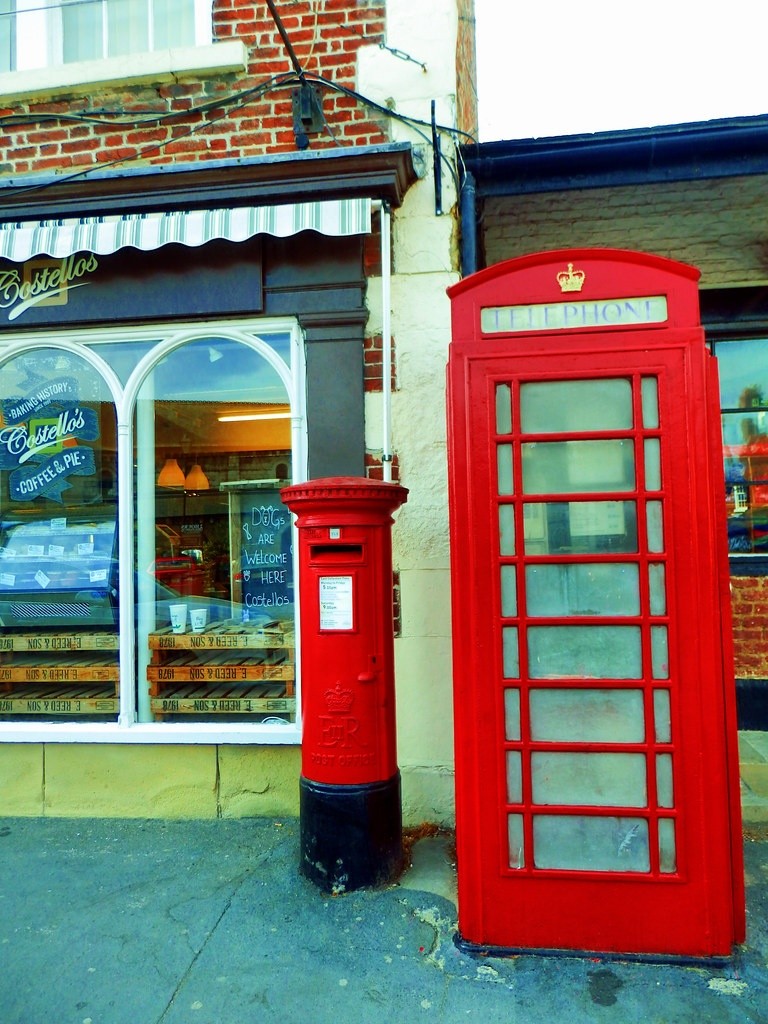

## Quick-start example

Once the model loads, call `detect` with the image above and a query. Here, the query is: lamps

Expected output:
[208,348,224,362]
[183,455,210,490]
[158,459,186,486]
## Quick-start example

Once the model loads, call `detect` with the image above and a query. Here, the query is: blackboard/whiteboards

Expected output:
[218,479,297,621]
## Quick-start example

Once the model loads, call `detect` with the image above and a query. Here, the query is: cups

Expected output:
[190,609,208,632]
[169,604,187,633]
